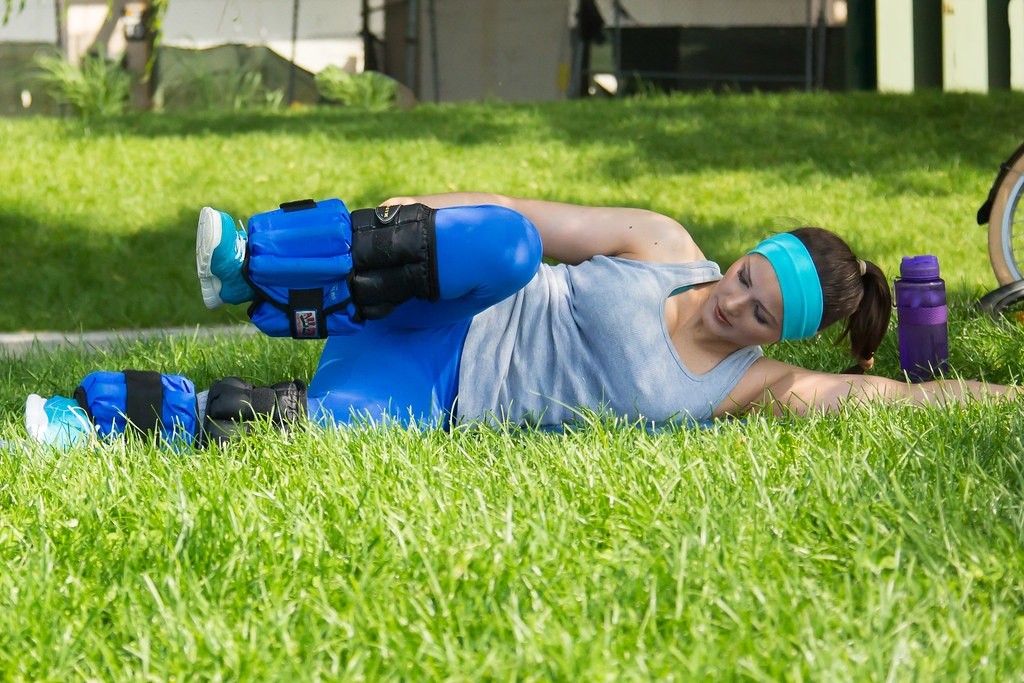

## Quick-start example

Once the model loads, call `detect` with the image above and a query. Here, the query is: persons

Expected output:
[24,194,1022,461]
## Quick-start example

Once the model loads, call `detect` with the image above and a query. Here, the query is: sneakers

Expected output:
[26,394,97,452]
[196,206,256,309]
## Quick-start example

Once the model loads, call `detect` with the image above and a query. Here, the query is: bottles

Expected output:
[895,255,950,382]
